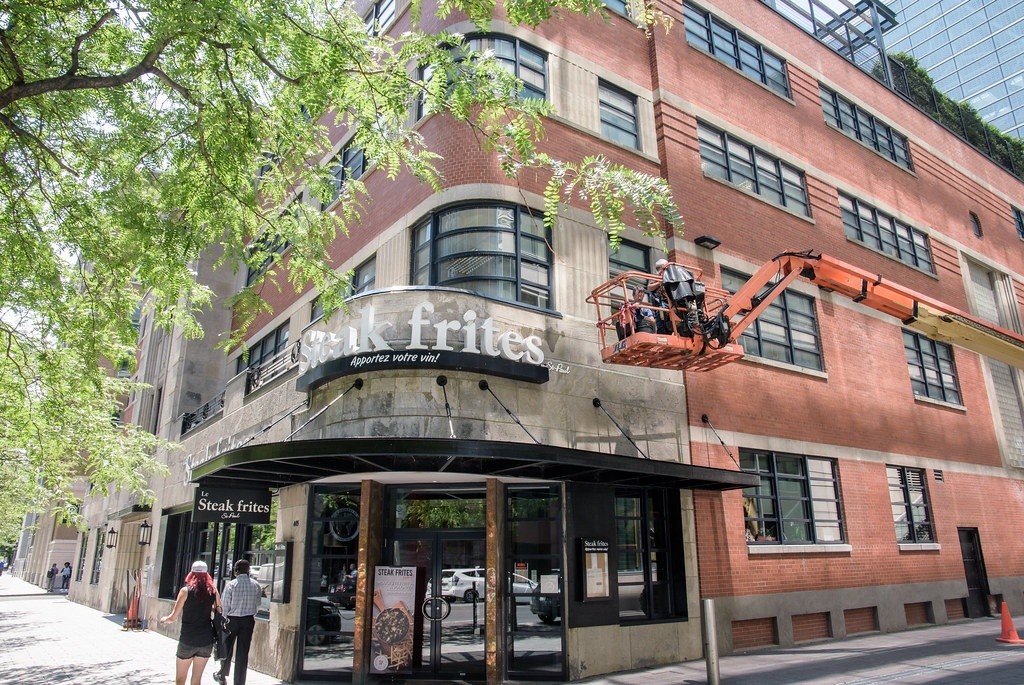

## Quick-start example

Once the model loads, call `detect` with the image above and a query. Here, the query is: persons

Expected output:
[61,562,72,592]
[160,561,222,685]
[345,563,357,588]
[616,260,699,340]
[213,560,261,685]
[320,575,328,592]
[47,564,58,592]
[0,560,4,576]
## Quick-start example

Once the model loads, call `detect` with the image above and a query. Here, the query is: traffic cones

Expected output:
[995,602,1024,644]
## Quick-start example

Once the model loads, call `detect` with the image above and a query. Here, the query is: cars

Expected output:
[204,549,274,619]
[439,566,540,605]
[327,579,357,610]
[529,573,562,625]
[306,597,342,647]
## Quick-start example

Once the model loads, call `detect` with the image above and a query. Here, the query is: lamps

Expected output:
[106,527,118,549]
[138,520,153,546]
[694,235,721,250]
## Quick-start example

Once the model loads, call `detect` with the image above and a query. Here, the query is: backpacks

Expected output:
[47,568,54,578]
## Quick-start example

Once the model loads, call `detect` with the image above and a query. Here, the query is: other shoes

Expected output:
[213,672,227,685]
[50,590,54,592]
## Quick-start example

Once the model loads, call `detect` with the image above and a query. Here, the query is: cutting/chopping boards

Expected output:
[373,588,414,655]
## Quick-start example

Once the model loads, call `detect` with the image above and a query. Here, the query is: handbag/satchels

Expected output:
[213,609,231,661]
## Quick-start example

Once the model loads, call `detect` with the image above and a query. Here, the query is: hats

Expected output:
[191,560,208,573]
